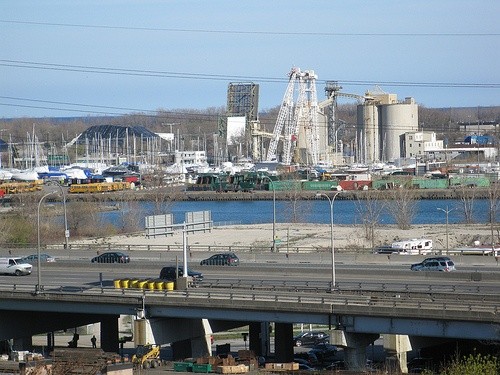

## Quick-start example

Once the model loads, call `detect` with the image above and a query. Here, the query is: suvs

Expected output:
[158,265,204,282]
[409,255,457,272]
[308,343,374,368]
[293,330,330,347]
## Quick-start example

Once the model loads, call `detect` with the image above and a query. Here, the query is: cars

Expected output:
[447,183,464,190]
[20,253,55,263]
[463,183,477,188]
[199,252,239,266]
[90,251,131,264]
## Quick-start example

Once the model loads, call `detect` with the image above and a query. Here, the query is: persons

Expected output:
[91,336,97,348]
[259,355,265,368]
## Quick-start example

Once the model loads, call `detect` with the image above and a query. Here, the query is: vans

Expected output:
[0,257,33,276]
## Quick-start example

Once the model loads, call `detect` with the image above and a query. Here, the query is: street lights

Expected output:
[37,190,58,291]
[316,190,347,288]
[47,180,69,249]
[258,176,277,254]
[436,206,459,256]
